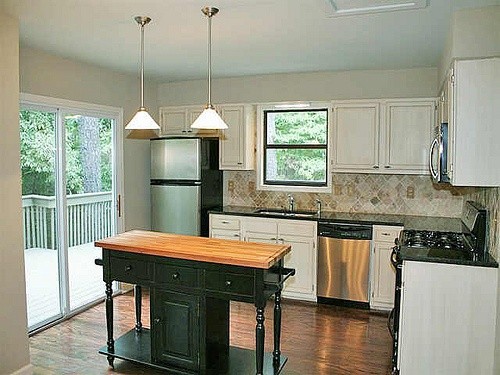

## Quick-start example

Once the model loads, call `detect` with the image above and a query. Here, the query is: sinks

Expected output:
[255,208,318,220]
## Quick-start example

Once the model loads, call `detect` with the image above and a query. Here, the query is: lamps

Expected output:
[123,16,162,130]
[190,6,229,130]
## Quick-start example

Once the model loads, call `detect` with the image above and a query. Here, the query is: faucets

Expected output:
[313,197,323,217]
[287,194,294,211]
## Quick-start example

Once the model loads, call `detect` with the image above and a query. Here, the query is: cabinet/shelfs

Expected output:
[158,103,256,171]
[370,225,404,311]
[94,229,296,375]
[438,57,500,187]
[332,98,439,176]
[209,215,319,306]
